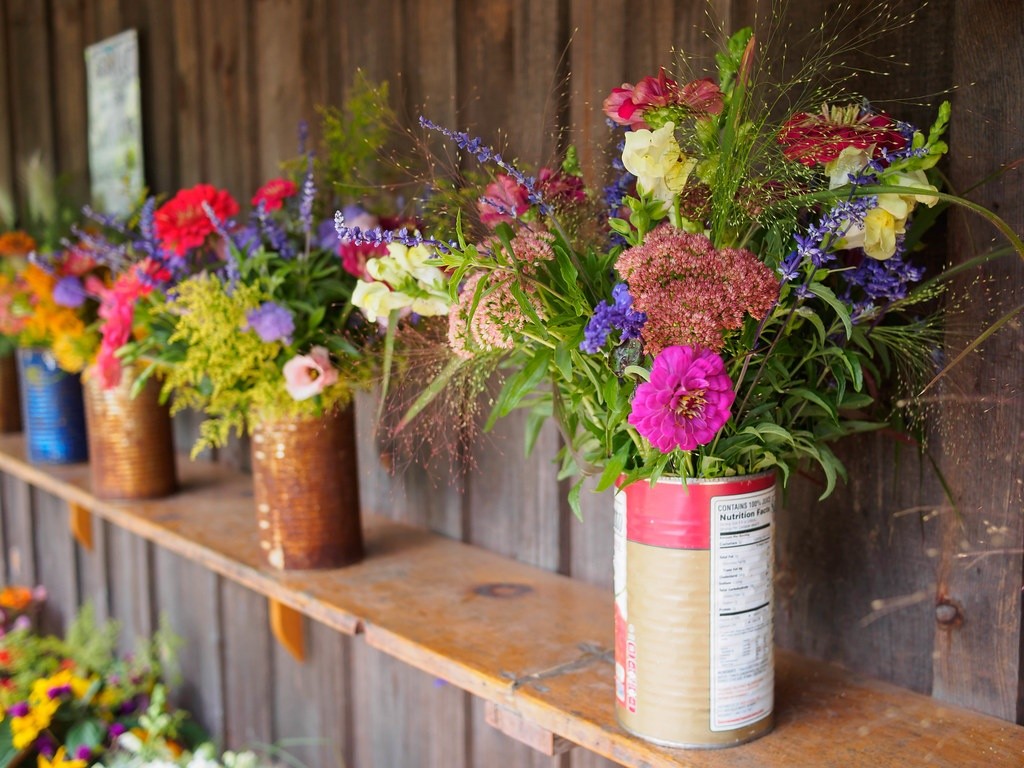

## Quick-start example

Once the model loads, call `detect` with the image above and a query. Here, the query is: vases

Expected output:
[78,342,179,501]
[612,463,778,746]
[237,398,366,571]
[16,343,87,467]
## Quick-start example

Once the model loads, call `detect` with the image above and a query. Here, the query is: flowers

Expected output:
[0,588,259,768]
[0,0,1023,516]
